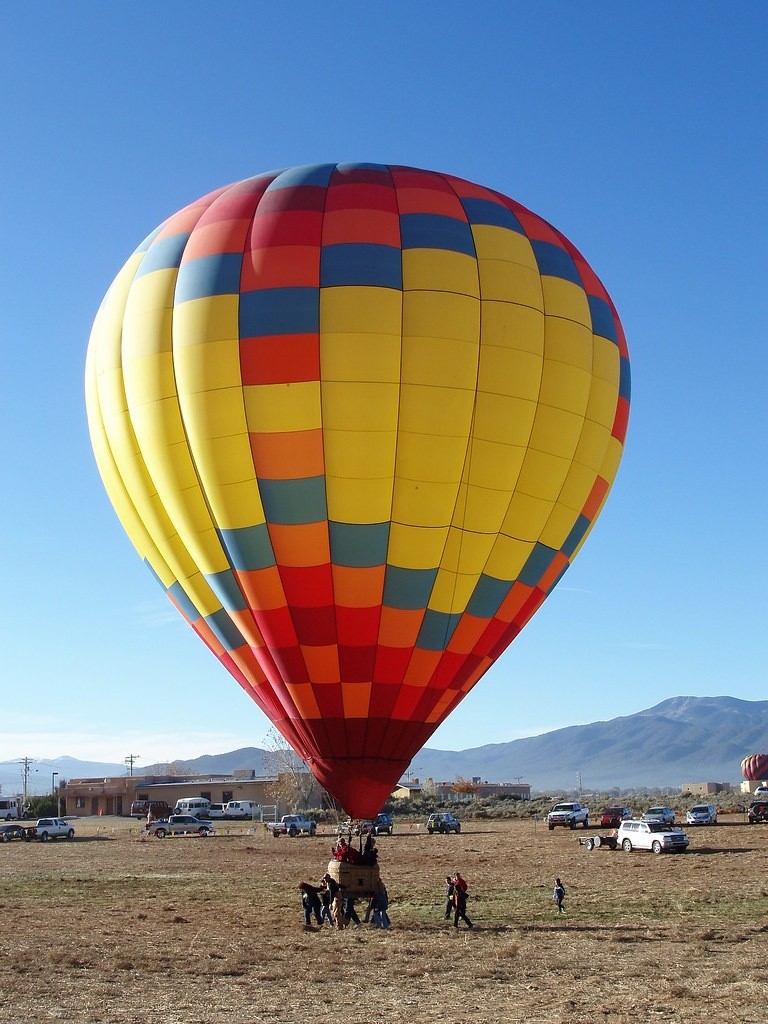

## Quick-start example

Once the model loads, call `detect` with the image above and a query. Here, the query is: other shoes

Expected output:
[373,924,380,928]
[562,907,565,913]
[380,926,387,929]
[557,911,561,915]
[353,923,363,929]
[362,920,369,923]
[468,923,473,928]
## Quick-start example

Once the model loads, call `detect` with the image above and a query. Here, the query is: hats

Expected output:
[323,874,330,879]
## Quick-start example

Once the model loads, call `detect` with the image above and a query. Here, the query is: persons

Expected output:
[297,834,394,930]
[445,872,473,930]
[552,877,566,914]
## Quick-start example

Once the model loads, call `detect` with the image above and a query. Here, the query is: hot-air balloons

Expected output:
[741,752,768,780]
[83,160,632,900]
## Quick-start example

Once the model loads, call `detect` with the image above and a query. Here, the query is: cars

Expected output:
[640,806,676,825]
[685,804,717,826]
[0,824,23,842]
[753,786,768,796]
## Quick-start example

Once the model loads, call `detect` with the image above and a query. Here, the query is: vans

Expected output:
[225,800,261,820]
[173,798,212,820]
[601,806,632,828]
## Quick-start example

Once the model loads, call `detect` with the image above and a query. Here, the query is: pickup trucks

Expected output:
[266,815,316,837]
[547,803,589,830]
[20,817,75,842]
[145,815,214,839]
[0,796,29,821]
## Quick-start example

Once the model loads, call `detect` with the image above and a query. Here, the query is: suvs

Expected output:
[208,802,228,820]
[617,819,689,854]
[747,800,768,824]
[426,812,461,834]
[355,813,393,836]
[130,800,173,821]
